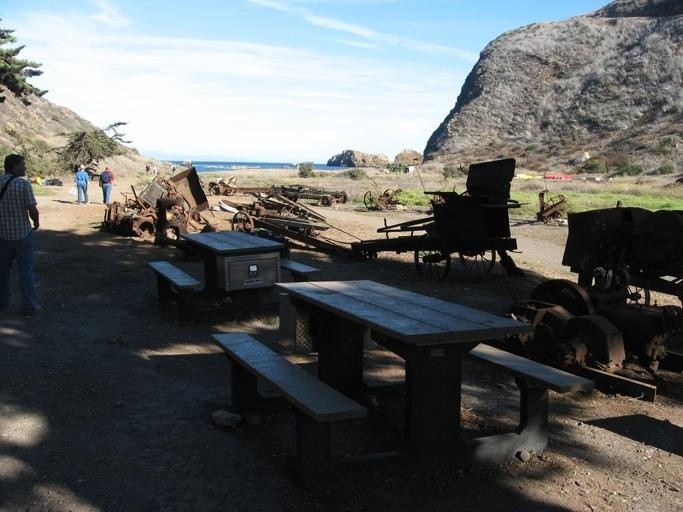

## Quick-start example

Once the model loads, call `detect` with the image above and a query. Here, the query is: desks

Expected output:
[274,278,537,468]
[177,230,285,318]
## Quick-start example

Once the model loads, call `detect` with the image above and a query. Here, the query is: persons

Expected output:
[145,162,150,176]
[0,154,41,313]
[97,166,116,206]
[72,163,89,204]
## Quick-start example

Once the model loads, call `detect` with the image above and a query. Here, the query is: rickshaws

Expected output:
[347,156,522,281]
[227,200,354,263]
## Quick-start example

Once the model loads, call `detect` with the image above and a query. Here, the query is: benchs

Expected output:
[210,331,368,481]
[147,258,200,321]
[469,341,594,462]
[279,257,322,281]
